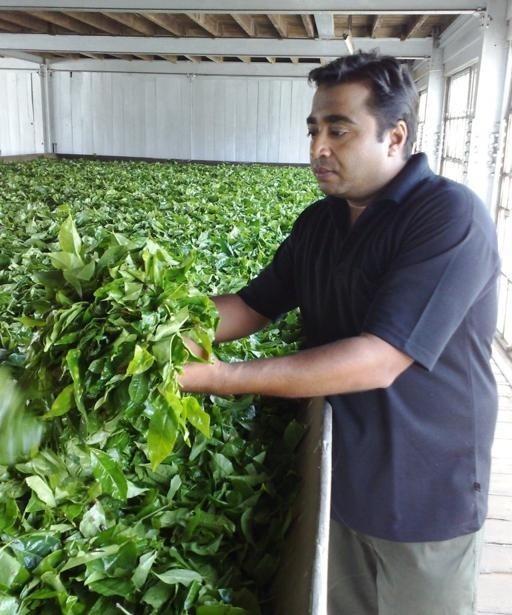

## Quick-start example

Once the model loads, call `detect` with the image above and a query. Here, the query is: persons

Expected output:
[174,48,501,615]
